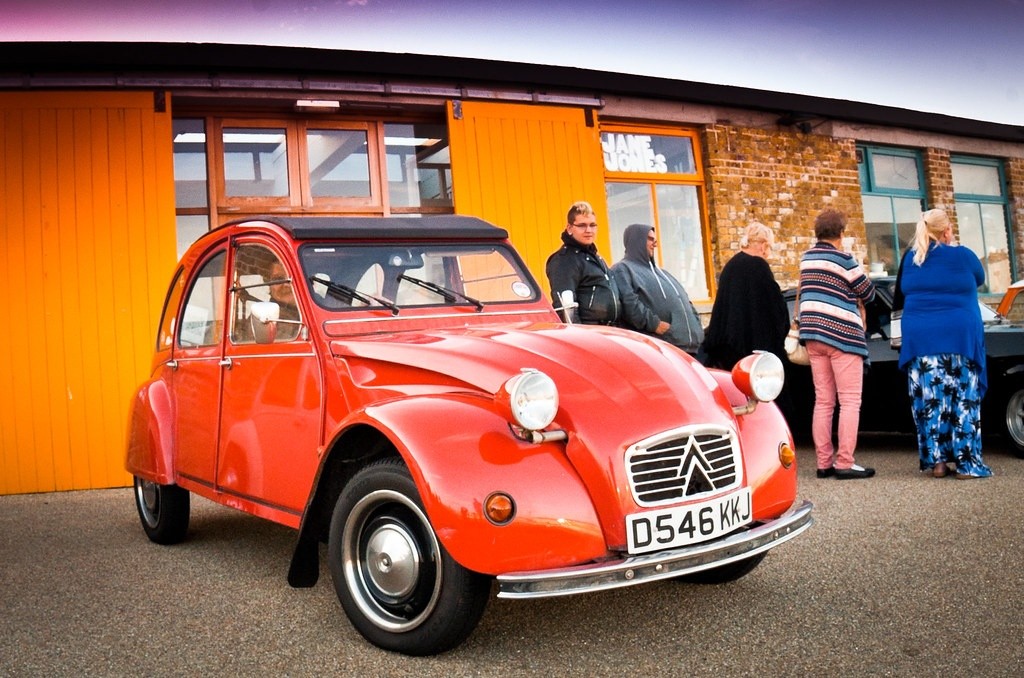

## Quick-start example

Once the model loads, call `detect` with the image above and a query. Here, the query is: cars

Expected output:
[123,216,815,657]
[700,274,1024,463]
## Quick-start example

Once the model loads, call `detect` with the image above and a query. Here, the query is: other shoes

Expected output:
[932,461,950,477]
[956,459,994,479]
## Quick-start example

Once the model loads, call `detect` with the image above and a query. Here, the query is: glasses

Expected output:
[573,223,598,228]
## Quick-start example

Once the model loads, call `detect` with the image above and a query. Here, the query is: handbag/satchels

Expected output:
[783,319,810,366]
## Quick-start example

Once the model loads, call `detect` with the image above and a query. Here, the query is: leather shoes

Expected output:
[817,466,836,478]
[835,466,876,480]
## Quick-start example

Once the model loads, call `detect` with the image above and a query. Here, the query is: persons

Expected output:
[242,258,300,341]
[898,209,995,480]
[610,223,705,358]
[799,209,875,479]
[695,222,804,392]
[546,202,622,327]
